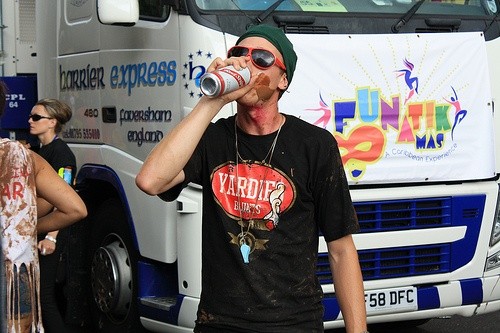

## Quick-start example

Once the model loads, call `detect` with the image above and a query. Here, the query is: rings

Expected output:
[42,248,46,256]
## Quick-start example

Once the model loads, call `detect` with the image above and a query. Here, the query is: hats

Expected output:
[235,24,298,101]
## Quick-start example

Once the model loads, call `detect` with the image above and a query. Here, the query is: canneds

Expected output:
[200,64,251,98]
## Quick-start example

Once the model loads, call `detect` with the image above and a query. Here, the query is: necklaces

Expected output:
[235,116,284,263]
[234,113,284,168]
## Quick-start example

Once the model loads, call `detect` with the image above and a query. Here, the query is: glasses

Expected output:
[28,114,54,121]
[227,46,286,70]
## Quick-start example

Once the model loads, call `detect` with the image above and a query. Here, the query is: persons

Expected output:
[28,98,77,333]
[135,24,367,333]
[0,79,87,333]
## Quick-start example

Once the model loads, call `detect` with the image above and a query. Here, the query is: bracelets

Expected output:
[46,234,57,244]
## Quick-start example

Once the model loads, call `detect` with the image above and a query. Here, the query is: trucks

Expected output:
[0,0,500,332]
